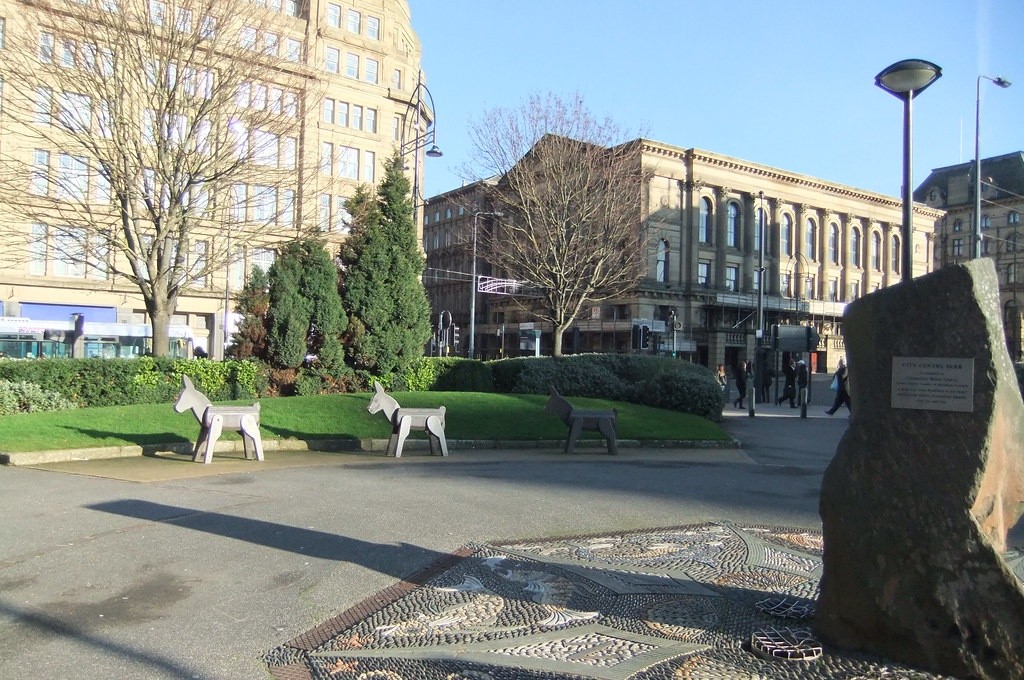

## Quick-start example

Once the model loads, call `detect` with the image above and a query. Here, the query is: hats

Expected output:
[797,360,804,364]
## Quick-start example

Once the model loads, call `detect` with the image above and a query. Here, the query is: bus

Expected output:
[0,316,194,364]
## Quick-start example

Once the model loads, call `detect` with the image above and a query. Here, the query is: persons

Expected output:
[762,366,772,403]
[824,356,850,417]
[778,359,797,408]
[797,360,812,407]
[734,358,755,409]
[716,364,727,391]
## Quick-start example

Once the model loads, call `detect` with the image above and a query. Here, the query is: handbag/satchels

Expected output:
[830,375,840,391]
[784,387,796,397]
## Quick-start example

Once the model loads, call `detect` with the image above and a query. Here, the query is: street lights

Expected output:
[873,57,944,282]
[399,65,443,238]
[469,210,504,360]
[972,73,1013,260]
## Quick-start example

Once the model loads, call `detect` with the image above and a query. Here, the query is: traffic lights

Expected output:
[639,324,651,350]
[451,323,460,346]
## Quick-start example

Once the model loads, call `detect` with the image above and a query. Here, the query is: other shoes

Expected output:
[824,410,833,415]
[767,399,769,403]
[733,401,736,407]
[777,398,781,406]
[739,406,746,409]
[790,406,797,408]
